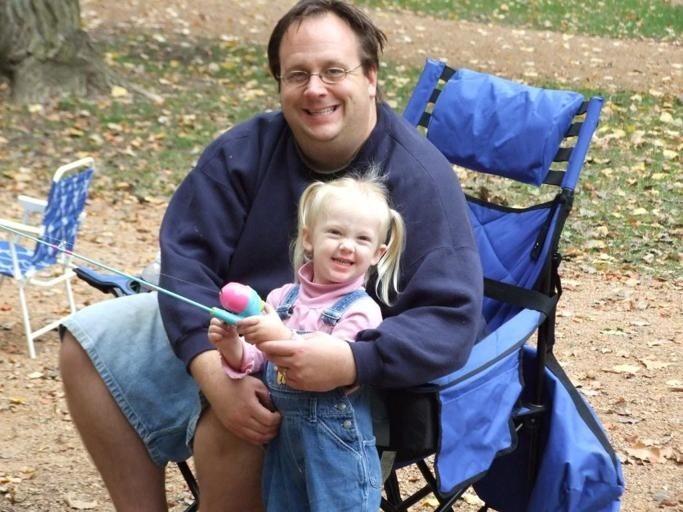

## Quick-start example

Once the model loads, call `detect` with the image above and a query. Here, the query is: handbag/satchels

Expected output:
[474,342,624,511]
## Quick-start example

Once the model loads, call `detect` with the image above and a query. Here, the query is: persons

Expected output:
[58,1,484,512]
[206,160,407,512]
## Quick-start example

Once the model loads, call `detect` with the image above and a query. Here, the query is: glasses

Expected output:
[274,63,363,84]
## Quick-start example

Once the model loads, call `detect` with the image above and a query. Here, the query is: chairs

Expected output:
[71,61,603,512]
[0,157,95,360]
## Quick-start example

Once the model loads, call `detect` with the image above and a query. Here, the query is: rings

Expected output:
[281,367,290,379]
